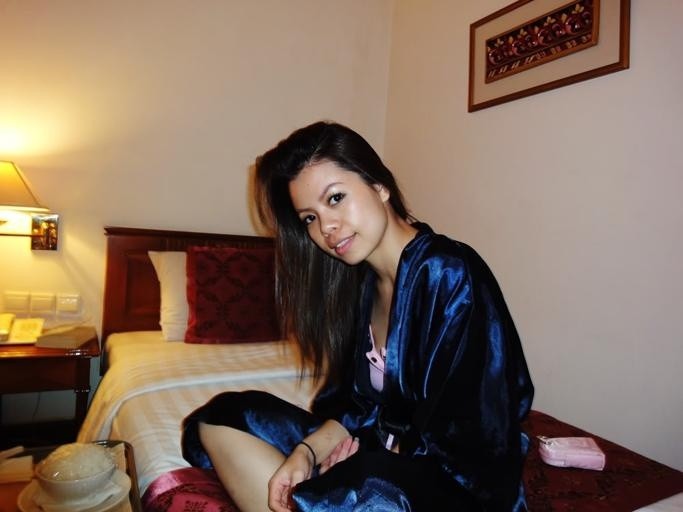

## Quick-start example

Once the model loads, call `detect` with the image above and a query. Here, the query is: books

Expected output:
[37,318,100,352]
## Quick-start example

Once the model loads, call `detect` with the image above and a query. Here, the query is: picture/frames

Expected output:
[467,0,631,113]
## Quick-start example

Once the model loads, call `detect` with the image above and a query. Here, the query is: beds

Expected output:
[98,224,682,510]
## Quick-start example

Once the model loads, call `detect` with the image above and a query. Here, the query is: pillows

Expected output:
[147,243,284,345]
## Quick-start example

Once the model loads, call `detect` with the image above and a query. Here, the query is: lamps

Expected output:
[0,160,58,251]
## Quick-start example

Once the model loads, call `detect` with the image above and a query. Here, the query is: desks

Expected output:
[0,327,101,447]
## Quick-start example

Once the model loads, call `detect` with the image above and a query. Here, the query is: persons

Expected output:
[181,118,536,512]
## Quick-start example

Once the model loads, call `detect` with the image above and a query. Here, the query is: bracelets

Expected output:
[291,438,321,469]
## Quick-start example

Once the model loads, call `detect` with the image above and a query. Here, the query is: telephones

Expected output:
[0,313,45,345]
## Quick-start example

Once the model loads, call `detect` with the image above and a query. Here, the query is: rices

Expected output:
[41,443,112,480]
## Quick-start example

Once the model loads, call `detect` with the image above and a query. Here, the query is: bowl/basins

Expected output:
[34,457,117,503]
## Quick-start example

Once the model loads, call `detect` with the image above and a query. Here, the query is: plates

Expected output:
[15,468,132,512]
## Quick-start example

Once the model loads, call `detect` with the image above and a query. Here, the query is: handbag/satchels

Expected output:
[535,435,605,472]
